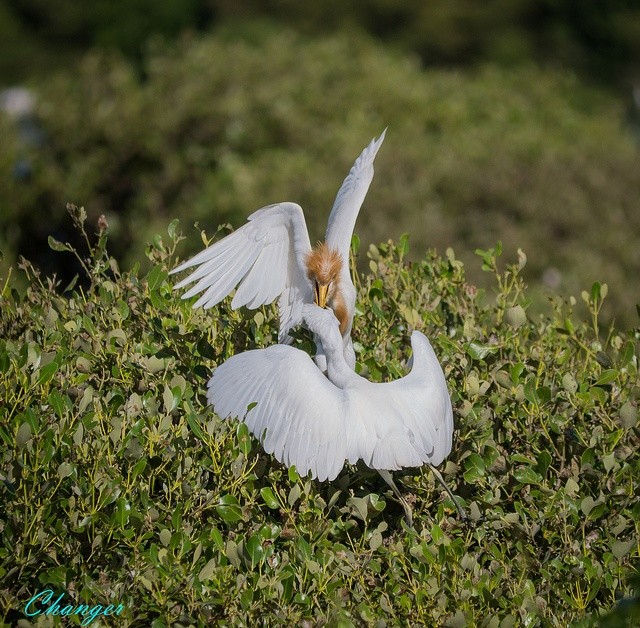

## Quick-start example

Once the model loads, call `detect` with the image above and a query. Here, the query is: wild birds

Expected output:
[167,125,389,378]
[204,299,469,546]
